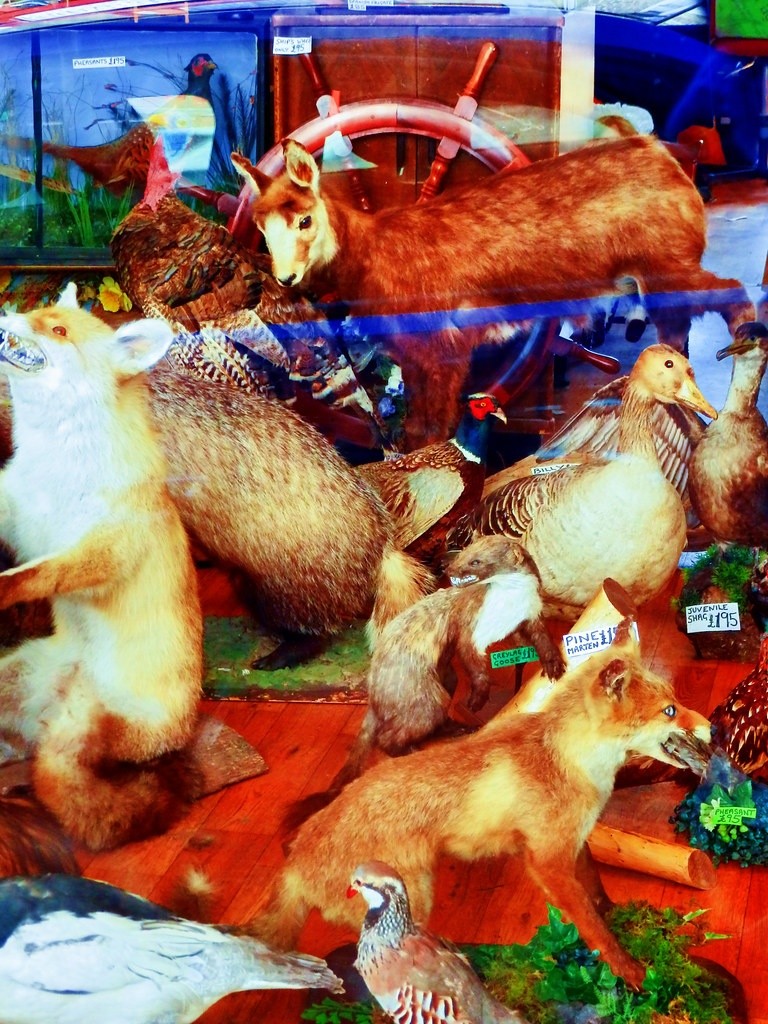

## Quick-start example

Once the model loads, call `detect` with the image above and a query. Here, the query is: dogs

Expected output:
[249,616,712,940]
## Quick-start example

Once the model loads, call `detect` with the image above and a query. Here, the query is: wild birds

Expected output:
[1,874,350,1023]
[350,860,519,1024]
[447,342,720,610]
[688,324,768,590]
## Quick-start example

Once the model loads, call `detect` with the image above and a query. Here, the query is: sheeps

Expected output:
[230,116,744,443]
